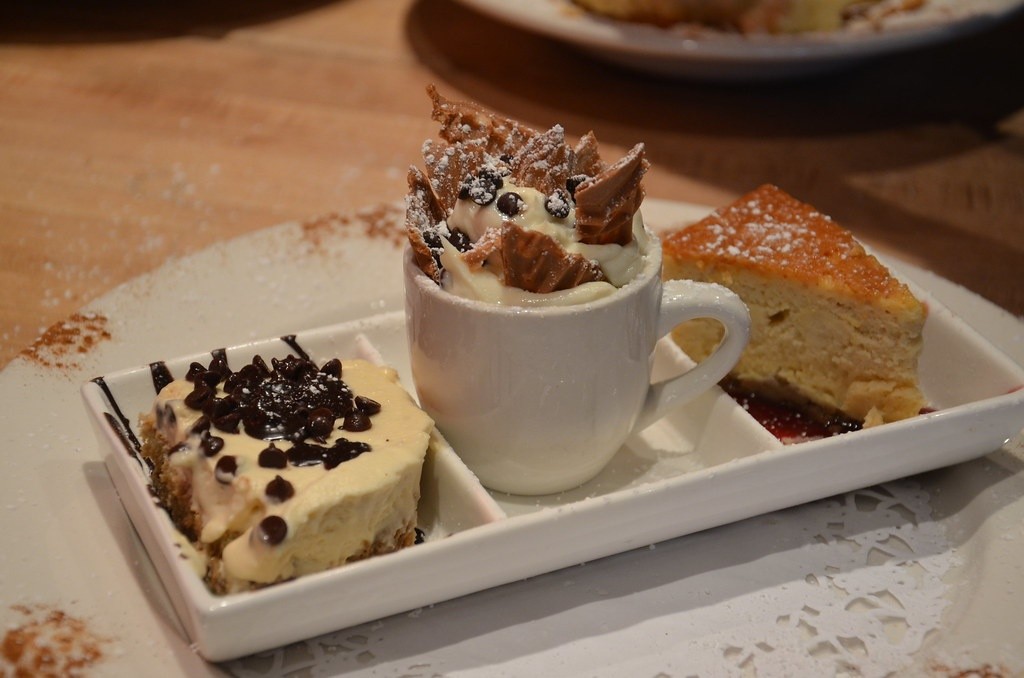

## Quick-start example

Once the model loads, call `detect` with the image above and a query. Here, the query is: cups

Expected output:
[405,225,749,493]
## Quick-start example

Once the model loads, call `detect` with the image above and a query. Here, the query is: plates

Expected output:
[1,198,1023,677]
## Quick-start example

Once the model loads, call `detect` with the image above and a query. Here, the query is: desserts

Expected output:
[653,182,927,433]
[139,357,437,595]
[405,85,655,308]
